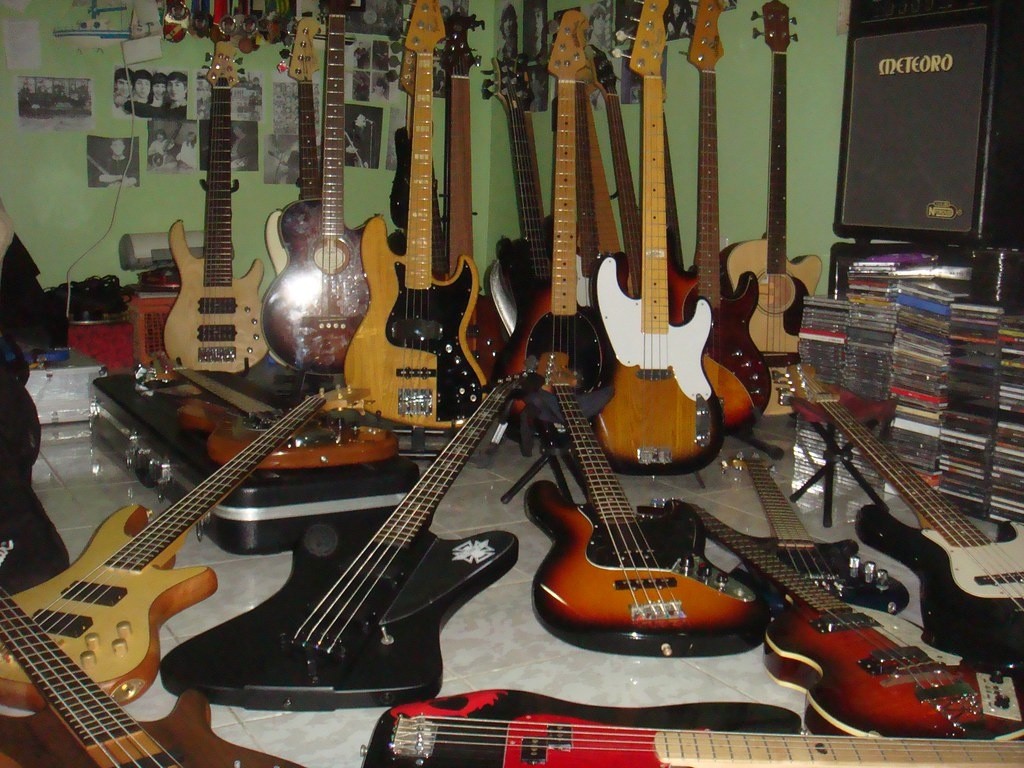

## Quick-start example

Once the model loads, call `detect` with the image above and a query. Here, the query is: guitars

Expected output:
[0,584,304,768]
[345,131,366,168]
[163,40,268,373]
[152,368,399,470]
[718,451,911,617]
[523,350,772,658]
[268,0,823,477]
[636,494,1024,742]
[151,130,176,169]
[158,370,520,713]
[359,688,1024,768]
[0,385,371,717]
[774,356,1024,672]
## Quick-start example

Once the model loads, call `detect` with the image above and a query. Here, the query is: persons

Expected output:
[149,129,177,168]
[523,3,548,61]
[587,4,609,50]
[666,2,690,40]
[114,68,187,118]
[231,123,251,170]
[528,73,545,112]
[176,132,197,168]
[354,41,369,68]
[197,72,262,121]
[274,82,319,133]
[493,3,517,62]
[375,43,389,70]
[20,80,89,118]
[344,114,374,168]
[371,80,390,102]
[354,78,369,101]
[94,139,138,186]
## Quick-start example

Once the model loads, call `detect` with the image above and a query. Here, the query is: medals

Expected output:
[160,0,297,54]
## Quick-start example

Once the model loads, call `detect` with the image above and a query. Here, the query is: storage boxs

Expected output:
[66,321,134,379]
[121,290,179,367]
